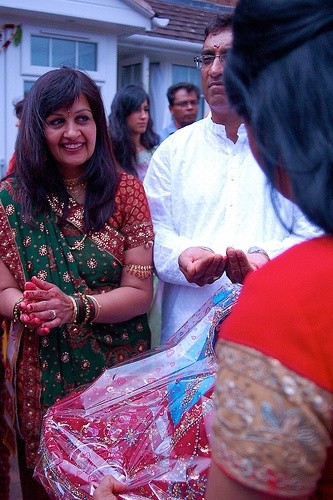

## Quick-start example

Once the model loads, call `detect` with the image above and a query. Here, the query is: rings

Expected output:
[213,276,220,281]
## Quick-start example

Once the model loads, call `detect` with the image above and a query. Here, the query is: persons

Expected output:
[141,10,324,351]
[0,64,157,500]
[33,0,333,500]
[14,80,200,188]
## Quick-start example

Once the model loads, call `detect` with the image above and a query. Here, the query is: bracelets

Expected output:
[12,296,25,326]
[247,247,270,260]
[67,291,102,327]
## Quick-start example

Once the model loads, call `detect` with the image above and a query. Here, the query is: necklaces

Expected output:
[63,178,85,193]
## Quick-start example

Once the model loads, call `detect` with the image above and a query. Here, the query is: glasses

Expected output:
[172,100,198,107]
[193,54,230,70]
